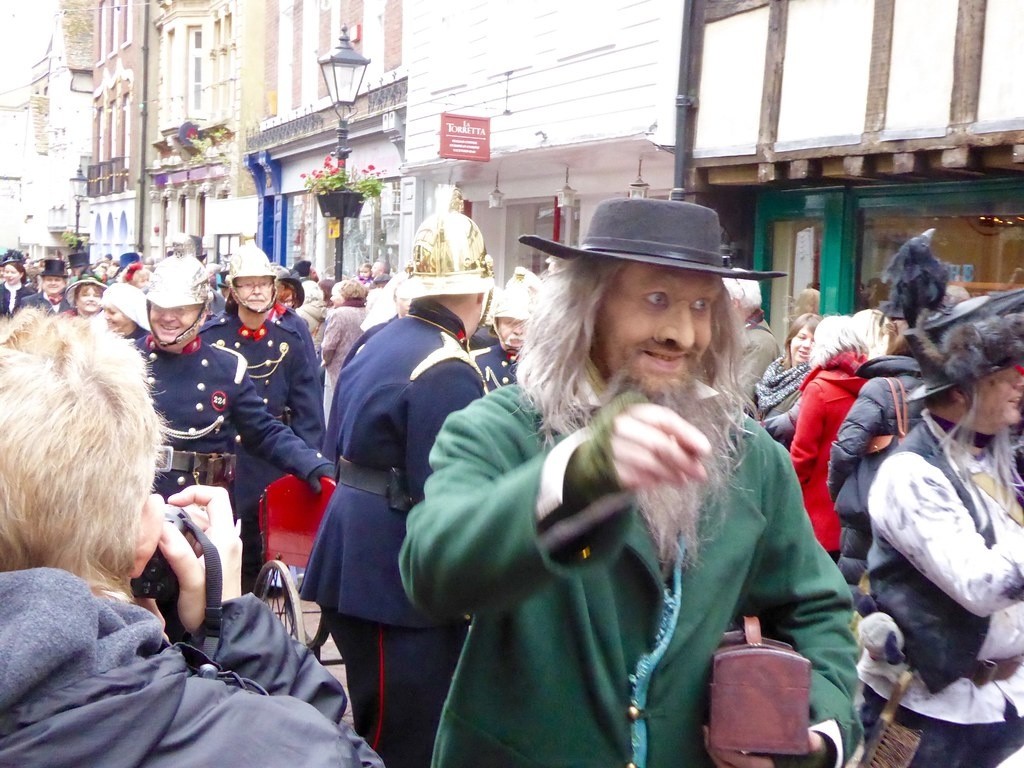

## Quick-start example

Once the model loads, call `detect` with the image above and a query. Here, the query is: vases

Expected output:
[316,190,366,218]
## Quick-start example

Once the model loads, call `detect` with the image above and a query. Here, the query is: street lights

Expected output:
[317,21,373,284]
[67,162,91,254]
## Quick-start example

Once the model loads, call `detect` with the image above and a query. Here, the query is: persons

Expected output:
[0,186,1024,768]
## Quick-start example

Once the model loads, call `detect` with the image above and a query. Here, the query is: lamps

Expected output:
[556,168,577,208]
[488,173,503,208]
[630,156,650,198]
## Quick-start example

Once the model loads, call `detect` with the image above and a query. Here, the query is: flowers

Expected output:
[299,155,385,198]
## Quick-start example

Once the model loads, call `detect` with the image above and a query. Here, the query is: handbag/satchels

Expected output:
[834,377,908,524]
[710,615,811,756]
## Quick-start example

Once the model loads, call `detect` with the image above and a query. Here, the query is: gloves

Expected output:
[309,463,341,494]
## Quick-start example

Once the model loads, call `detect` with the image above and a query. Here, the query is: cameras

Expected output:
[126,500,190,599]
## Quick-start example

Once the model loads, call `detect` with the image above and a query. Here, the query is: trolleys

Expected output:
[249,474,344,666]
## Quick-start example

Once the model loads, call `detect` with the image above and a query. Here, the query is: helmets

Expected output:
[226,233,277,283]
[493,266,538,321]
[145,233,213,309]
[396,188,494,298]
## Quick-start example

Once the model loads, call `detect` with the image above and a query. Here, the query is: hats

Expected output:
[118,252,140,270]
[66,252,93,269]
[65,277,108,306]
[0,251,20,267]
[520,198,787,280]
[41,259,68,277]
[273,264,305,308]
[881,237,1024,403]
[294,261,312,277]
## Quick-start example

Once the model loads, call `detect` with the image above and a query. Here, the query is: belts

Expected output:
[156,449,236,485]
[962,657,1024,685]
[339,455,414,511]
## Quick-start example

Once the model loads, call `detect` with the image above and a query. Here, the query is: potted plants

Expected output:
[61,229,88,250]
